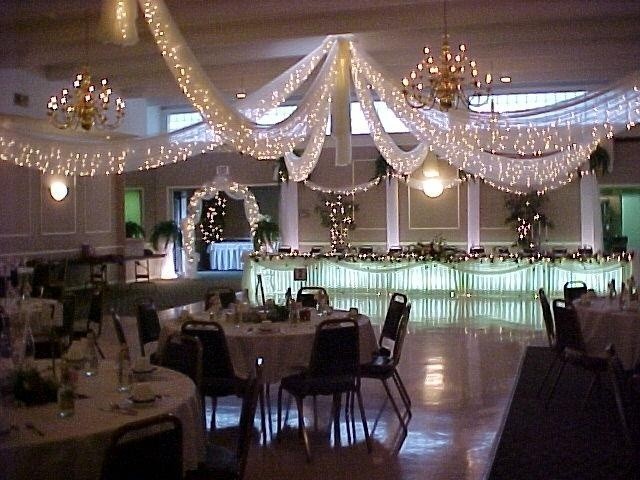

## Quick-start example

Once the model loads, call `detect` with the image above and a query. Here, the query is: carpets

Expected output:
[485,344,640,480]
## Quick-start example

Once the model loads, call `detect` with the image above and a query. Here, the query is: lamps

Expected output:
[51,180,67,201]
[424,148,441,177]
[402,41,495,115]
[47,73,124,132]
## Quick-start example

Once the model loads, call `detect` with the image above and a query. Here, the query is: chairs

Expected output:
[205,286,237,308]
[577,245,594,259]
[493,247,511,256]
[181,319,269,462]
[565,280,587,310]
[388,247,401,254]
[200,366,261,479]
[311,244,322,255]
[162,329,202,389]
[295,286,332,310]
[347,292,412,414]
[278,318,372,463]
[279,245,292,257]
[470,247,486,258]
[105,415,182,480]
[355,303,412,433]
[552,245,567,259]
[136,297,162,355]
[110,309,132,362]
[537,287,557,349]
[607,345,640,446]
[536,300,624,414]
[29,295,72,358]
[68,301,105,360]
[359,245,372,254]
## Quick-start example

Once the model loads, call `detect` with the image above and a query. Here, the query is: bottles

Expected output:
[65,340,83,395]
[241,289,250,322]
[58,367,75,425]
[84,334,96,376]
[13,333,26,367]
[610,278,615,300]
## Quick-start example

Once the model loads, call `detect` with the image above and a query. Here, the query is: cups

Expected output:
[130,381,151,400]
[133,353,151,369]
[259,319,272,333]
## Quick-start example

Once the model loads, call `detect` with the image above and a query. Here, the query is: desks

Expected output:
[61,255,167,288]
